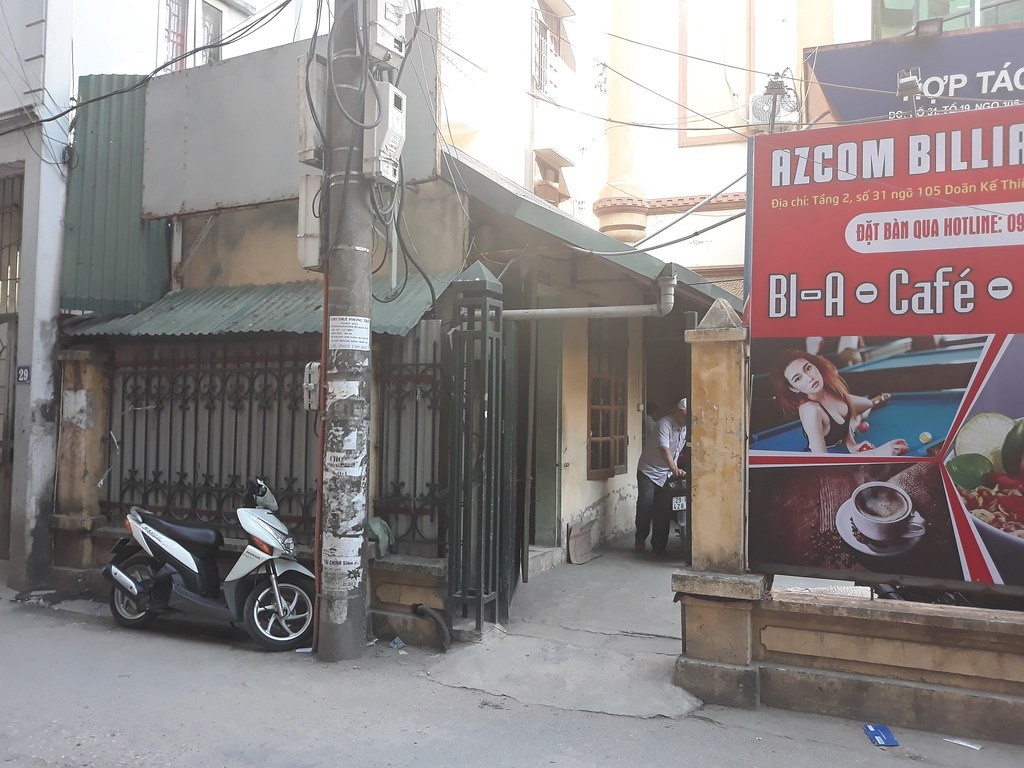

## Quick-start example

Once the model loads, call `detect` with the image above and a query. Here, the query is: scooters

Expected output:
[100,474,317,652]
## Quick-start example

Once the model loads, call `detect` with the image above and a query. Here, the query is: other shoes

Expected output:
[653,545,666,556]
[635,537,645,553]
[870,392,891,409]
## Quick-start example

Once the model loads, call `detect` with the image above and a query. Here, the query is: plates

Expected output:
[836,499,922,555]
[943,415,1024,548]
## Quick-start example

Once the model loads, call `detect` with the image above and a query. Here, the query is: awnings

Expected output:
[63,267,462,336]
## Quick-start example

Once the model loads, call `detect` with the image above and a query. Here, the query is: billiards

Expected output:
[859,421,870,433]
[858,444,872,453]
[919,431,933,444]
[896,440,909,454]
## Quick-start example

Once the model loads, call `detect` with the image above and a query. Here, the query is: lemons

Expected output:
[945,412,1024,489]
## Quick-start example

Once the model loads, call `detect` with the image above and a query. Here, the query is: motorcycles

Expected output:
[668,473,692,564]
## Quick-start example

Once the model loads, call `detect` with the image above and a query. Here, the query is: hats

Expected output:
[677,397,686,410]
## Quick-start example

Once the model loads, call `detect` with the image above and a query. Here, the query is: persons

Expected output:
[772,350,906,456]
[635,398,686,558]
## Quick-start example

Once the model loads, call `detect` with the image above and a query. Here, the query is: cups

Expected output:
[850,482,926,542]
